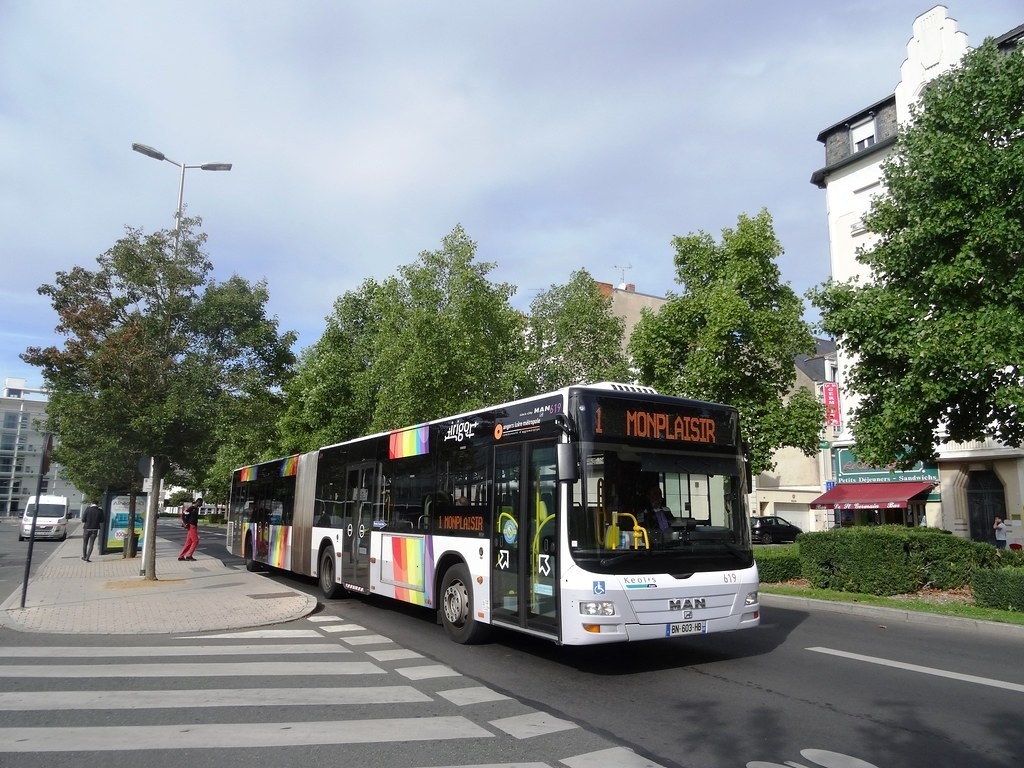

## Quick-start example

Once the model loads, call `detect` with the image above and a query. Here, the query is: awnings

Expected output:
[810,483,931,509]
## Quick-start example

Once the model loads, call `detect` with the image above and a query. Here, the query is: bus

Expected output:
[226,382,762,653]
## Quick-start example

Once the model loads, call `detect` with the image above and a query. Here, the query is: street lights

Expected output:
[126,138,235,576]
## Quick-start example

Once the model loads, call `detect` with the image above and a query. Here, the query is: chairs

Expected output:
[540,493,553,516]
[422,491,452,530]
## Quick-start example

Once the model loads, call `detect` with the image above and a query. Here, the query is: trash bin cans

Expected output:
[123,533,141,558]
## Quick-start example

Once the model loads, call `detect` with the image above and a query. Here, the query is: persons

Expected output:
[81,500,104,563]
[638,485,675,531]
[177,498,203,561]
[993,515,1006,550]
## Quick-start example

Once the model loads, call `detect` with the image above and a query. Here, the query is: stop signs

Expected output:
[41,435,54,475]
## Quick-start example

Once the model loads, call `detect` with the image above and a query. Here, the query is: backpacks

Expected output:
[181,506,195,529]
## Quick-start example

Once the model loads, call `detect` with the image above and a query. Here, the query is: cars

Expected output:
[747,516,805,544]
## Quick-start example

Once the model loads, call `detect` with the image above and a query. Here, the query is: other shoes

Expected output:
[186,557,196,561]
[178,557,187,561]
[82,557,85,560]
[86,559,91,562]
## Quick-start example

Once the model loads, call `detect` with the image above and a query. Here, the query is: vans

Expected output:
[18,494,72,542]
